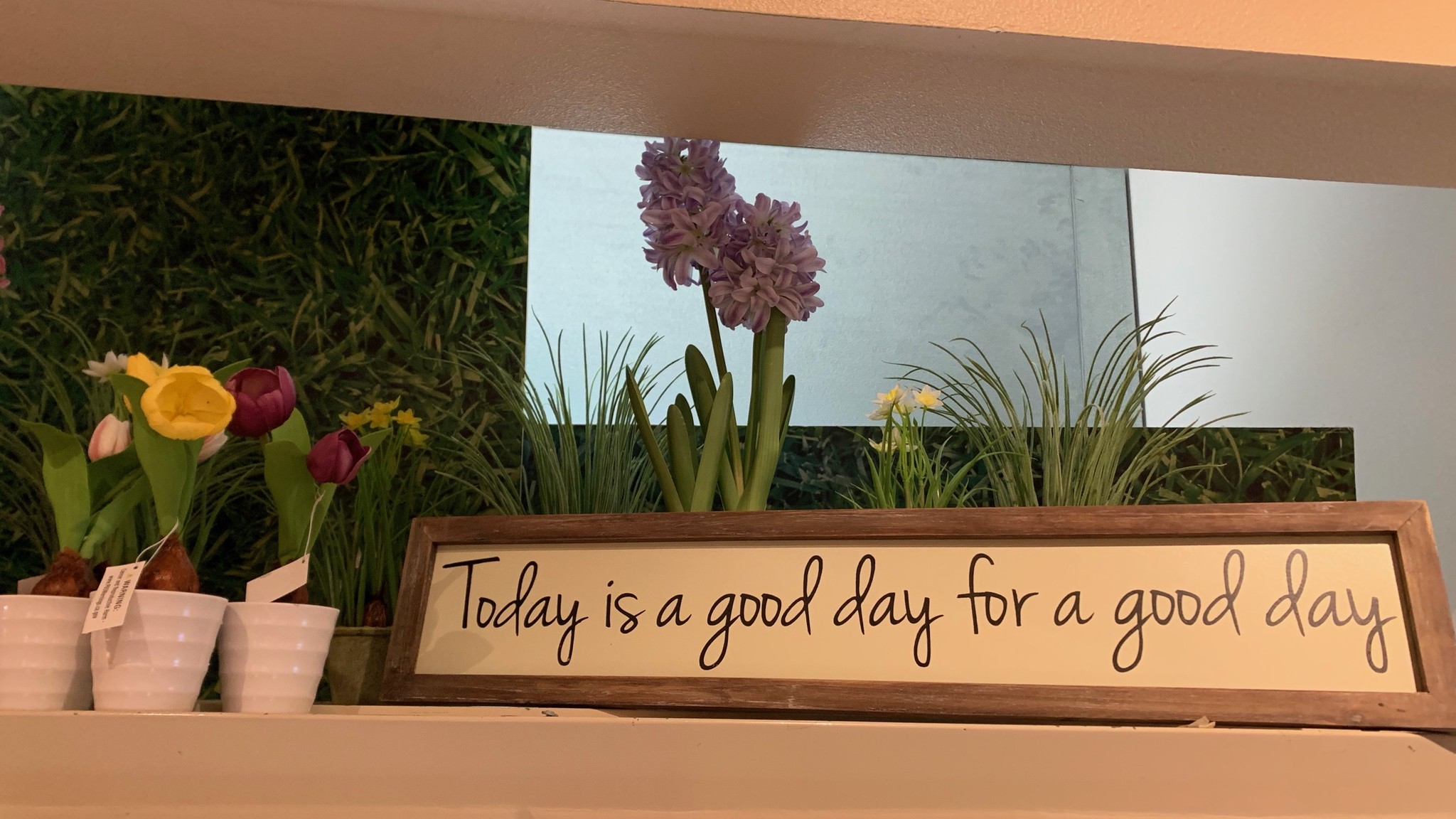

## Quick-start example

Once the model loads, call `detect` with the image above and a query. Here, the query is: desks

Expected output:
[0,684,1456,819]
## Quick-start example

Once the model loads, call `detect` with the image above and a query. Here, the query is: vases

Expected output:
[88,586,228,714]
[0,593,92,712]
[217,600,341,716]
[323,625,392,706]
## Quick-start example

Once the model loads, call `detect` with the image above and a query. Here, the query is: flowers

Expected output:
[866,385,948,508]
[18,347,433,593]
[621,133,832,513]
